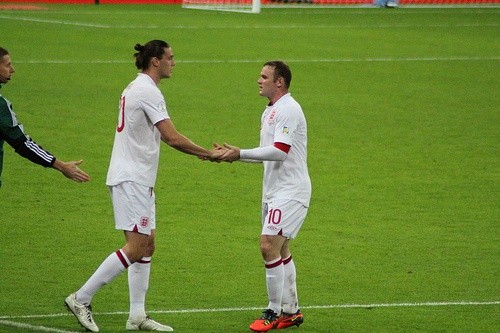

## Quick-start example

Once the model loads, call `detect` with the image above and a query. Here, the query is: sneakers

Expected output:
[275,311,304,330]
[64,292,100,333]
[249,309,284,332]
[125,316,174,332]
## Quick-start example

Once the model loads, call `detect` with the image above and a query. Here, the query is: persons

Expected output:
[199,61,311,333]
[0,46,90,191]
[65,40,228,333]
